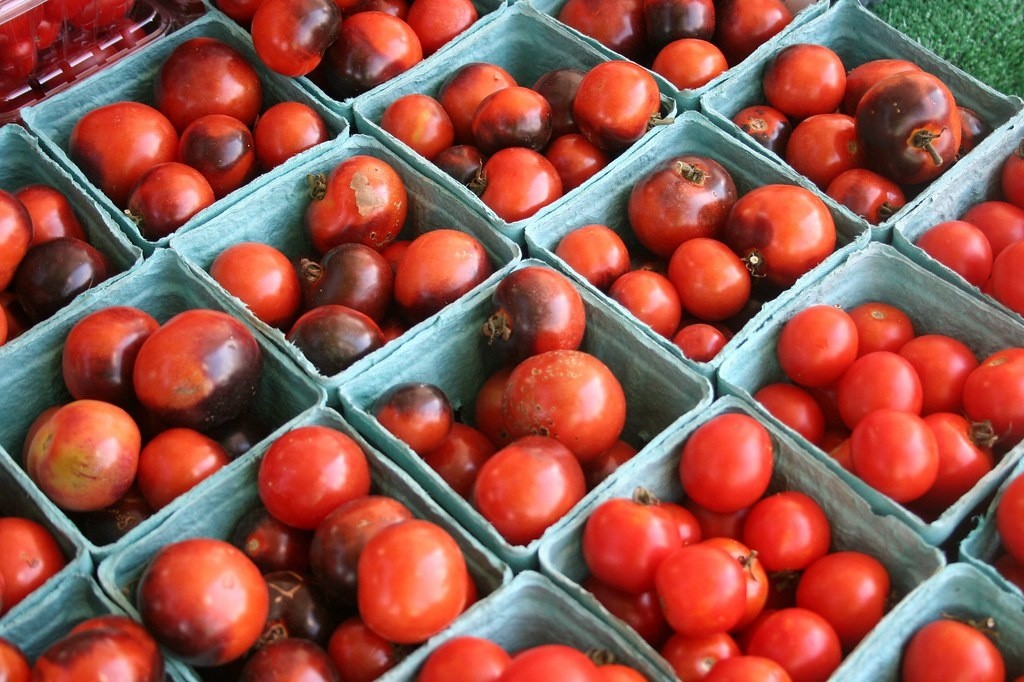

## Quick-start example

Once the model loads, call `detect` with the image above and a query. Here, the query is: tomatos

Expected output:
[0,0,1024,682]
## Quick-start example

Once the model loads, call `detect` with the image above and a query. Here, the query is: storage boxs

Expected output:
[0,0,1024,682]
